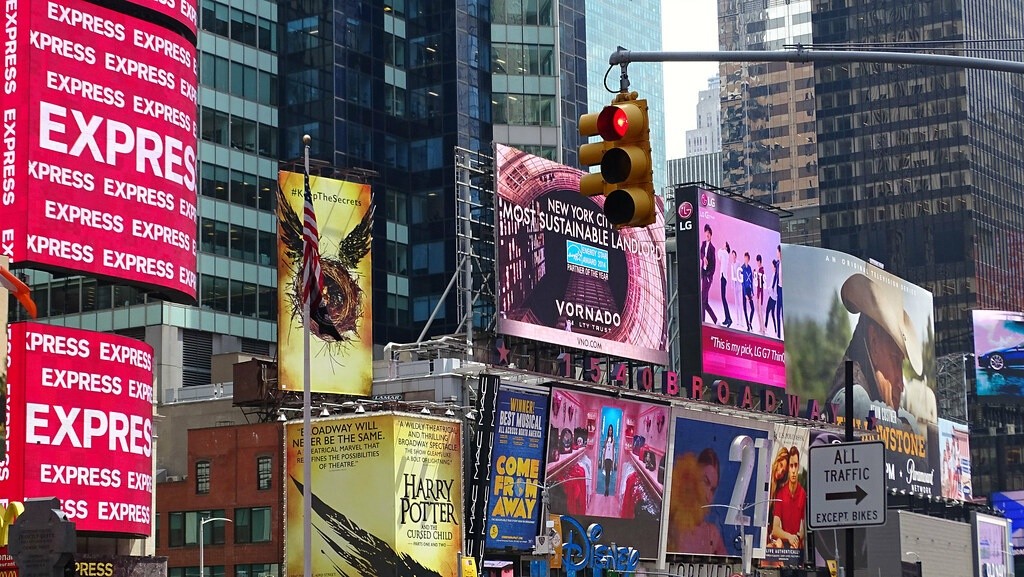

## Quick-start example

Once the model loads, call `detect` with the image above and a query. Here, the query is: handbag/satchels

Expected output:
[643,450,656,471]
[548,423,560,463]
[639,443,650,462]
[574,427,588,447]
[632,434,646,456]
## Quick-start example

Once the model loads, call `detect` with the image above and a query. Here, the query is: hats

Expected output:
[841,263,923,376]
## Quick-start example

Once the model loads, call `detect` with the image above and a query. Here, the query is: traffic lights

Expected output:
[576,111,607,213]
[597,97,657,228]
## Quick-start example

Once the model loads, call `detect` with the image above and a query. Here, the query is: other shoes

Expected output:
[722,319,733,328]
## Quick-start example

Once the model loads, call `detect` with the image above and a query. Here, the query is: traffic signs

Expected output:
[807,439,889,530]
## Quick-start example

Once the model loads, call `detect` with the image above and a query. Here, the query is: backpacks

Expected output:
[657,452,666,485]
[559,428,573,454]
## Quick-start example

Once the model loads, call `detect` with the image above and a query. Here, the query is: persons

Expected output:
[699,224,785,341]
[820,263,924,435]
[942,435,963,500]
[765,445,806,552]
[321,285,330,308]
[600,425,615,497]
[665,448,729,554]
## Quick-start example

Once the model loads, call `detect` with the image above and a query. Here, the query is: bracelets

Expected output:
[796,535,800,542]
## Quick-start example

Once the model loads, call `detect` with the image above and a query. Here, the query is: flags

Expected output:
[300,166,346,342]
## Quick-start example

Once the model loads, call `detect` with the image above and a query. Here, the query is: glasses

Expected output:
[952,435,954,437]
